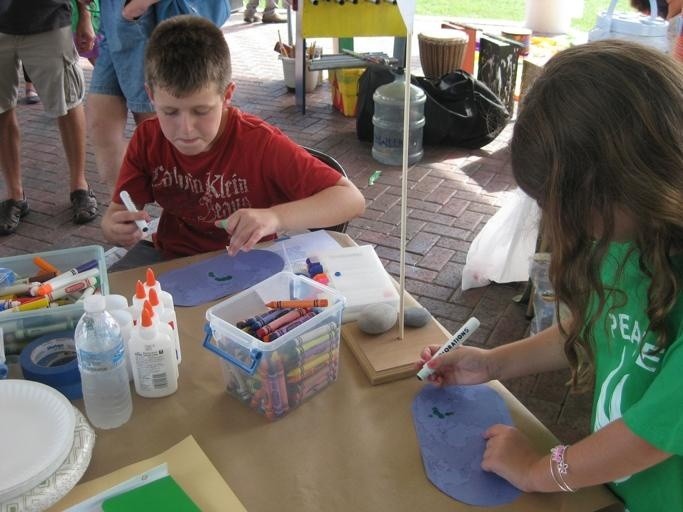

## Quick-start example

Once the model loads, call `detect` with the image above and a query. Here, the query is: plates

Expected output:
[0,380,75,500]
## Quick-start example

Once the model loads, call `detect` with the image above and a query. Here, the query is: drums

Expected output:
[502,27,533,55]
[418,29,468,82]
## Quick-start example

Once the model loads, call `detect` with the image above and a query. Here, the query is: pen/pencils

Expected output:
[217,299,340,419]
[119,189,149,233]
[416,317,481,382]
[0,257,102,359]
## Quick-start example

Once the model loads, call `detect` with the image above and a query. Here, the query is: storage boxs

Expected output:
[0,244,110,365]
[200,271,349,421]
[326,67,368,117]
[522,252,559,336]
[278,46,321,94]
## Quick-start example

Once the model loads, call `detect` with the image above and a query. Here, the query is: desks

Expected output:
[0,226,623,511]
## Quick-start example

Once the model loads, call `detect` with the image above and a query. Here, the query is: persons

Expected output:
[23,65,40,104]
[100,15,367,262]
[0,0,96,235]
[243,0,288,23]
[630,0,683,20]
[71,0,103,66]
[417,39,683,512]
[85,0,231,205]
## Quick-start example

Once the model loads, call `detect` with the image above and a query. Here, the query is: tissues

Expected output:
[527,1,584,34]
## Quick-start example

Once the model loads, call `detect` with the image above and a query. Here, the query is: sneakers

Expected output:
[244,14,260,23]
[262,13,287,23]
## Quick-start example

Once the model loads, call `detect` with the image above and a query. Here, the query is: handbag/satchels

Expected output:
[357,64,509,149]
[154,0,232,29]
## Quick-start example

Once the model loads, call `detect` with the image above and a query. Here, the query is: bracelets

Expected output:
[550,444,574,492]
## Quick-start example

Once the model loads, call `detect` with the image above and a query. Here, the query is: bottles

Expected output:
[73,266,180,429]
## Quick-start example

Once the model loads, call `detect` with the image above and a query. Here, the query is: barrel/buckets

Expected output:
[371,67,427,168]
[280,54,322,93]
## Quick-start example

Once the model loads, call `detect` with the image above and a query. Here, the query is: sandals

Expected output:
[0,192,29,236]
[70,185,98,223]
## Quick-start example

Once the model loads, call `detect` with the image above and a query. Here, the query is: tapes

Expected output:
[20,330,83,400]
